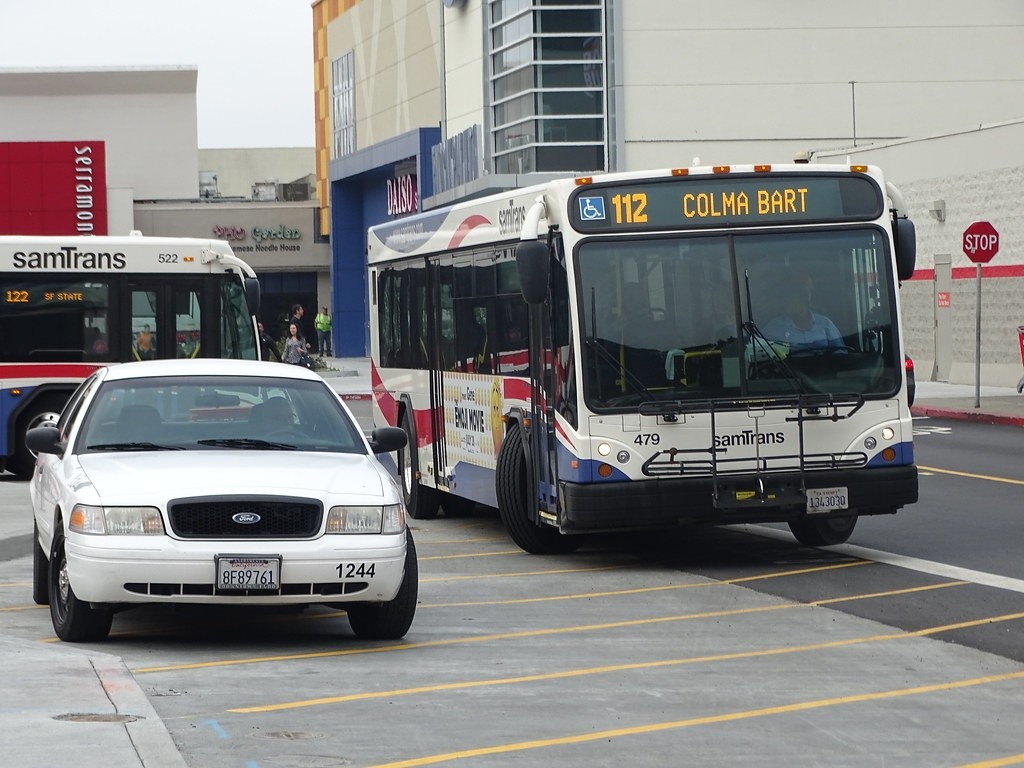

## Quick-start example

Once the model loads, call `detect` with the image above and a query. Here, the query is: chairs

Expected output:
[117,405,162,444]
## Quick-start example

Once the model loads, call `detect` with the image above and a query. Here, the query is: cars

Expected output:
[24,359,419,644]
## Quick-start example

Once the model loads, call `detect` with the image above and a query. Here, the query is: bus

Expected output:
[1,228,261,482]
[368,161,918,555]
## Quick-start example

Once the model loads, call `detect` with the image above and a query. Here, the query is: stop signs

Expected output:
[965,218,1000,264]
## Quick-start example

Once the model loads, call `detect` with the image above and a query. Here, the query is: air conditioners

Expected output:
[276,184,311,201]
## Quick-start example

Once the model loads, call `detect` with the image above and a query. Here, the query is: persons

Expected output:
[501,322,523,350]
[280,305,311,365]
[258,396,311,446]
[314,304,333,358]
[134,323,157,361]
[759,273,849,358]
[603,282,654,332]
[257,322,283,363]
